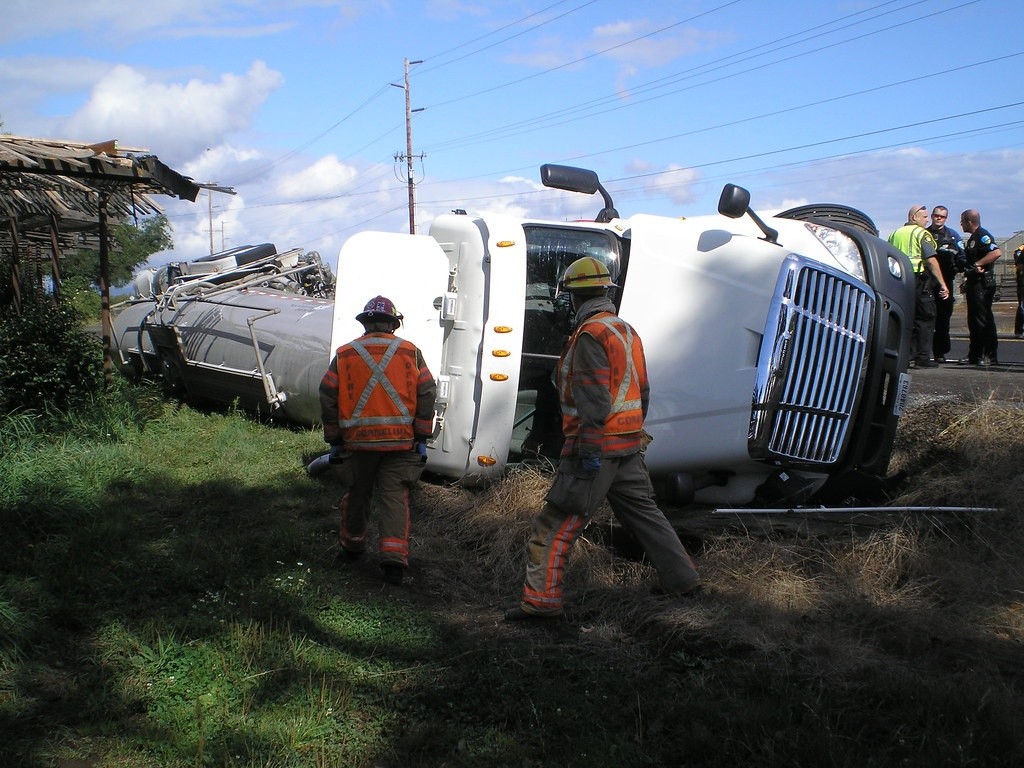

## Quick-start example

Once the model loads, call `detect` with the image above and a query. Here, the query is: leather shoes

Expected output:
[959,355,980,363]
[934,354,945,362]
[979,357,997,365]
[914,360,938,369]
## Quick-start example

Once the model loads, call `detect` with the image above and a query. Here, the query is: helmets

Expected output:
[355,295,401,329]
[554,257,621,300]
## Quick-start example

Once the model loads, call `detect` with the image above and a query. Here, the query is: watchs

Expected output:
[972,263,983,268]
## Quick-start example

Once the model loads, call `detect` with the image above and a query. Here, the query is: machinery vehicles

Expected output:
[107,162,916,514]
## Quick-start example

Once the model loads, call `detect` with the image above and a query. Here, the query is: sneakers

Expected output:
[381,563,404,586]
[504,607,564,623]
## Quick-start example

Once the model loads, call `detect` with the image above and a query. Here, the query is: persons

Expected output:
[922,206,967,366]
[315,292,439,586]
[888,205,952,368]
[501,252,704,626]
[951,208,1004,367]
[1011,242,1024,340]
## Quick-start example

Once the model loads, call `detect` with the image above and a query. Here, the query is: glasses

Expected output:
[914,205,926,215]
[932,214,947,219]
